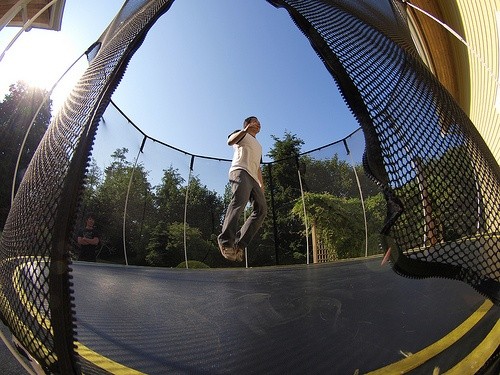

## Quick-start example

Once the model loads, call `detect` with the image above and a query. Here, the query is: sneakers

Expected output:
[217,234,237,262]
[234,243,244,262]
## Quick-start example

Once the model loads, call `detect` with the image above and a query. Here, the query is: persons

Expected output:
[76,214,102,264]
[216,116,268,262]
